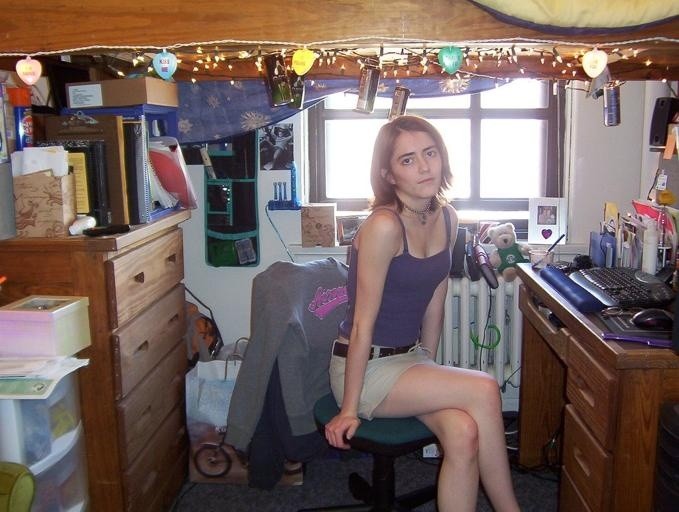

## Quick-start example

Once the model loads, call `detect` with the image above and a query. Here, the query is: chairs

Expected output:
[252,257,437,512]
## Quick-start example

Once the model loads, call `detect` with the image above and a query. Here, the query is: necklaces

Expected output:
[404,202,431,225]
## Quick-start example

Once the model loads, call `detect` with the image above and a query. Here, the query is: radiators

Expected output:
[438,276,522,412]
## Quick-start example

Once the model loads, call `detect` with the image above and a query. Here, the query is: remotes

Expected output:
[82,224,130,237]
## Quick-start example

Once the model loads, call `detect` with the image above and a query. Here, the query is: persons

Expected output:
[259,124,294,170]
[546,208,555,224]
[324,116,518,511]
[539,208,548,224]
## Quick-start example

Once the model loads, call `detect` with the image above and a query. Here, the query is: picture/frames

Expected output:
[528,197,568,244]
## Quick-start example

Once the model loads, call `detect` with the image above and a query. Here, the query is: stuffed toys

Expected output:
[487,222,534,283]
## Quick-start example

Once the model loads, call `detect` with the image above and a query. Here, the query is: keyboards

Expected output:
[568,266,677,307]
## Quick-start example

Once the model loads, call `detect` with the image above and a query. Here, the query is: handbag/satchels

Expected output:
[185,360,303,486]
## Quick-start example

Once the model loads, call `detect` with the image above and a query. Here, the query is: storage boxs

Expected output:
[65,76,179,109]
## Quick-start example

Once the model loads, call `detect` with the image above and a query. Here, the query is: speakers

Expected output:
[649,97,679,146]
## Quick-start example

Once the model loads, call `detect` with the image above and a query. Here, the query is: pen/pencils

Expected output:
[602,333,674,348]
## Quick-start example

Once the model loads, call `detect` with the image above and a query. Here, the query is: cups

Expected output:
[528,250,555,269]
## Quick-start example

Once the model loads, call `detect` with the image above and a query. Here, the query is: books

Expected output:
[11,112,199,238]
[299,201,337,248]
[449,229,472,279]
[1,355,90,402]
[594,199,678,269]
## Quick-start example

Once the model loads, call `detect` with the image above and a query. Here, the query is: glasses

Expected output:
[601,306,644,319]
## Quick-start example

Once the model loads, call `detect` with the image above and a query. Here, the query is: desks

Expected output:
[516,263,679,512]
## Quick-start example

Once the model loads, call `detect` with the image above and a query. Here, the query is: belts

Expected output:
[333,342,416,361]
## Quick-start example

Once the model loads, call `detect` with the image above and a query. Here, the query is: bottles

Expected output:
[641,221,659,277]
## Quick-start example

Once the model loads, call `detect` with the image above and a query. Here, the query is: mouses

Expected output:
[631,309,675,330]
